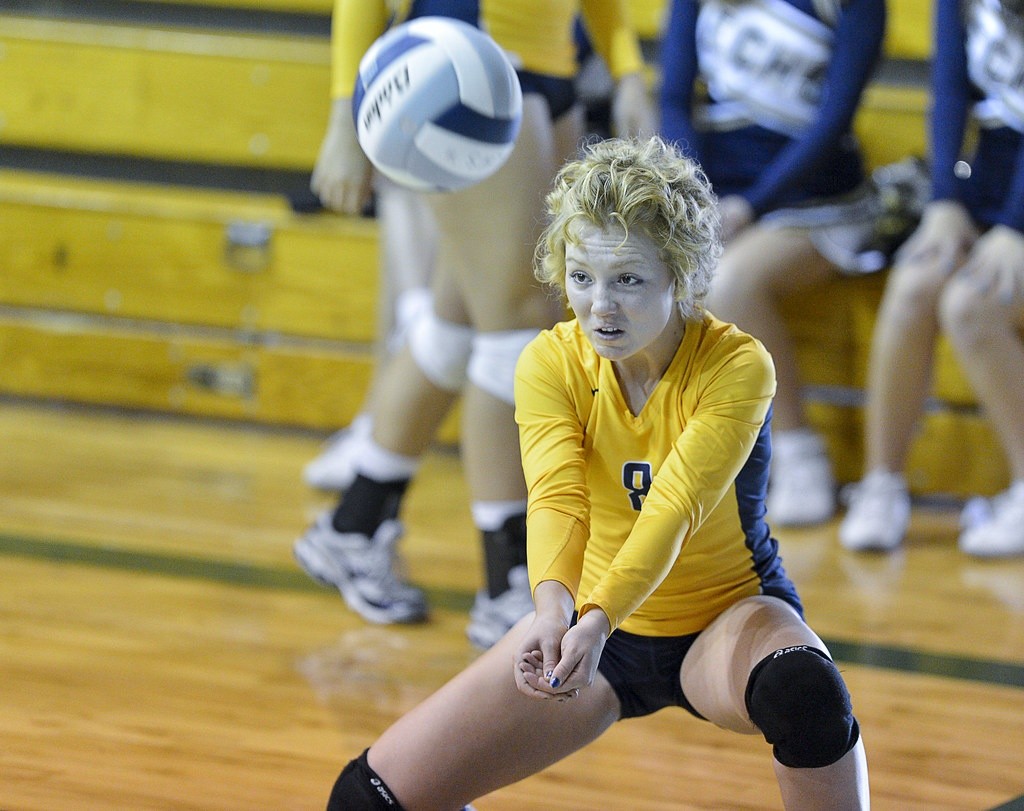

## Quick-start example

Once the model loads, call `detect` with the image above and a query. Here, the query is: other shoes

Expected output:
[832,473,913,546]
[956,479,1024,558]
[766,430,837,526]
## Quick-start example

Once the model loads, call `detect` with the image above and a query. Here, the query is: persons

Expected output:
[288,2,1024,646]
[321,135,874,811]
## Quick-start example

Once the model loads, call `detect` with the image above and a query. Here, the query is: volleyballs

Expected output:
[348,10,527,199]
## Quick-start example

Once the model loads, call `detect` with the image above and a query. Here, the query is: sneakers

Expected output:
[290,508,427,624]
[468,565,539,650]
